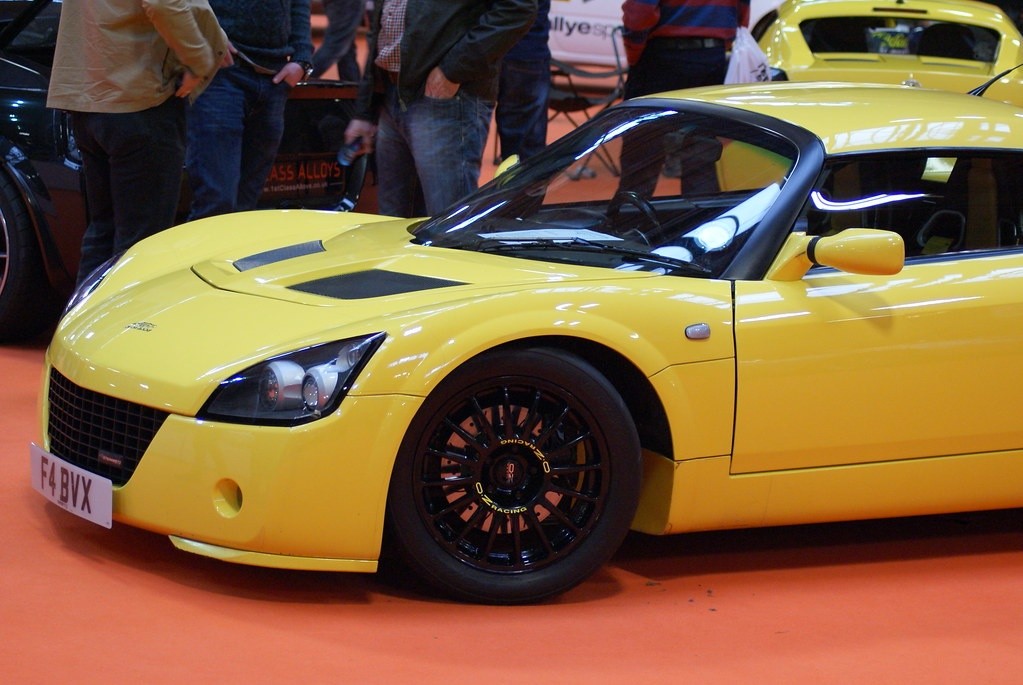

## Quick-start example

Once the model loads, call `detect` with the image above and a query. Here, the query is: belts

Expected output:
[645,37,725,51]
[370,65,399,86]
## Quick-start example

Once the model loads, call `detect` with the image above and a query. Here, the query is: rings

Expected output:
[187,90,191,93]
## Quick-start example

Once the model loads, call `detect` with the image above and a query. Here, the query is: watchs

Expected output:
[291,59,314,83]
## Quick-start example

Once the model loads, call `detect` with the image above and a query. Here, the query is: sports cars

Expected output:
[31,82,1023,604]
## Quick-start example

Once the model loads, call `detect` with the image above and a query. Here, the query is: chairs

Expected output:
[825,156,885,238]
[547,57,619,184]
[915,208,968,254]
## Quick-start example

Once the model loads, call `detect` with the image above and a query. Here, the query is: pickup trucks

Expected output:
[0,0,367,340]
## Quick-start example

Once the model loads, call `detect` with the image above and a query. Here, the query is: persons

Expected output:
[46,0,554,290]
[611,0,750,201]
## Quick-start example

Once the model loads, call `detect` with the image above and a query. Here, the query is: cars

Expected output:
[547,0,1023,193]
